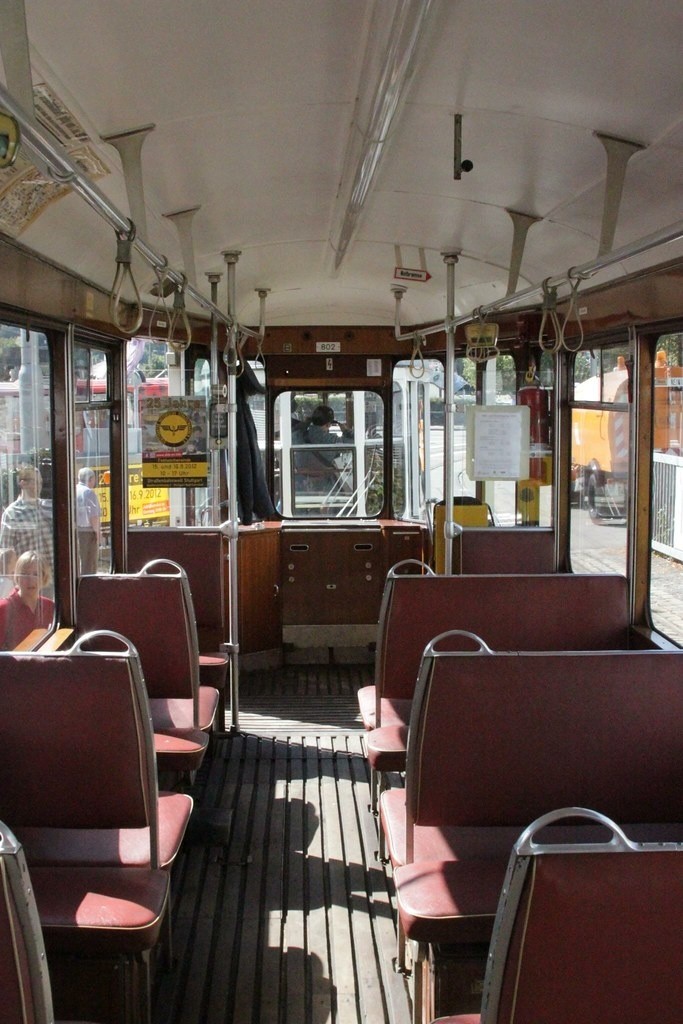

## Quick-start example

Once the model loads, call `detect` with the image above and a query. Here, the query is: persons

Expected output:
[0,465,54,602]
[76,467,100,575]
[0,551,55,652]
[0,548,17,600]
[291,406,355,514]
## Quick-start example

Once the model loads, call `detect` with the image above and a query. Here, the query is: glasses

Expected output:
[19,477,43,484]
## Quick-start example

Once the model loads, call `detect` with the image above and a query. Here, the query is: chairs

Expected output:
[0,559,229,1024]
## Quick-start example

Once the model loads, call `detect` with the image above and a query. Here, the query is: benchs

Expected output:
[358,558,683,1024]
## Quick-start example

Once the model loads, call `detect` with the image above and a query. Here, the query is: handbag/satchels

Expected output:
[330,460,352,497]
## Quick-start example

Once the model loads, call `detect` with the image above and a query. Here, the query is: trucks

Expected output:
[570,350,683,521]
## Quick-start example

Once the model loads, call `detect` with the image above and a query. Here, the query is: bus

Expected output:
[0,379,211,528]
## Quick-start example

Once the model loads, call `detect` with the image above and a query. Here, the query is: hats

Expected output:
[312,406,339,423]
